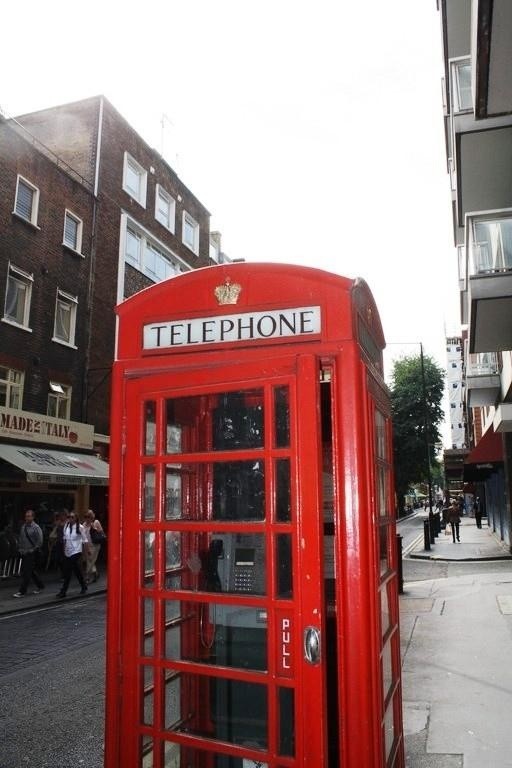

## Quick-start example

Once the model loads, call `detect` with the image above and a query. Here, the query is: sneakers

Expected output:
[12,591,26,598]
[79,585,88,595]
[32,588,46,595]
[92,571,101,583]
[55,592,67,599]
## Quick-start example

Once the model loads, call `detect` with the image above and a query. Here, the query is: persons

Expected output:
[447,499,461,543]
[12,510,45,598]
[471,497,483,529]
[49,507,104,598]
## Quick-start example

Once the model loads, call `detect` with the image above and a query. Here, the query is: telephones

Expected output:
[210,532,269,628]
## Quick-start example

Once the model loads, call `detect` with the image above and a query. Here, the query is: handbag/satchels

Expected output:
[89,519,105,545]
[34,549,44,575]
